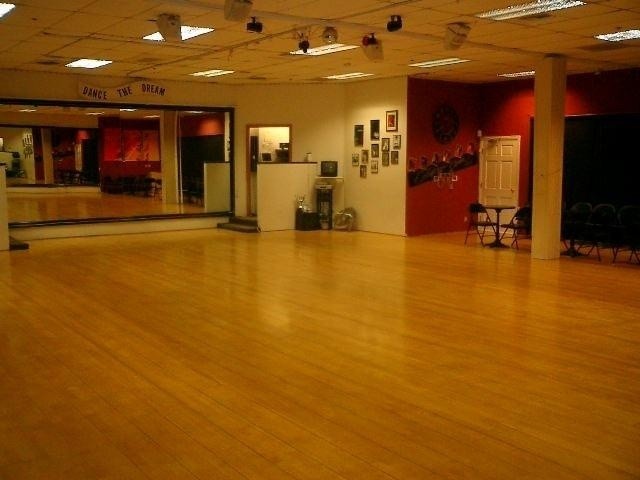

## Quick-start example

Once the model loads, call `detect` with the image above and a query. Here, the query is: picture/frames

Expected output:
[349,109,401,180]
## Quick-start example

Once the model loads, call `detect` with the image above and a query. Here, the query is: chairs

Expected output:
[465,202,640,263]
[102,173,204,207]
[58,168,98,187]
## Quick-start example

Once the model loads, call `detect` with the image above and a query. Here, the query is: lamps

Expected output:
[233,15,407,66]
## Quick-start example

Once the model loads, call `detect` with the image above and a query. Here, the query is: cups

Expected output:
[306,152,313,162]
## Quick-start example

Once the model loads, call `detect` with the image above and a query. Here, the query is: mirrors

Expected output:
[0,98,236,228]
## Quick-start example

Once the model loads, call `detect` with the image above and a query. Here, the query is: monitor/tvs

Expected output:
[321,161,337,177]
[260,152,273,162]
